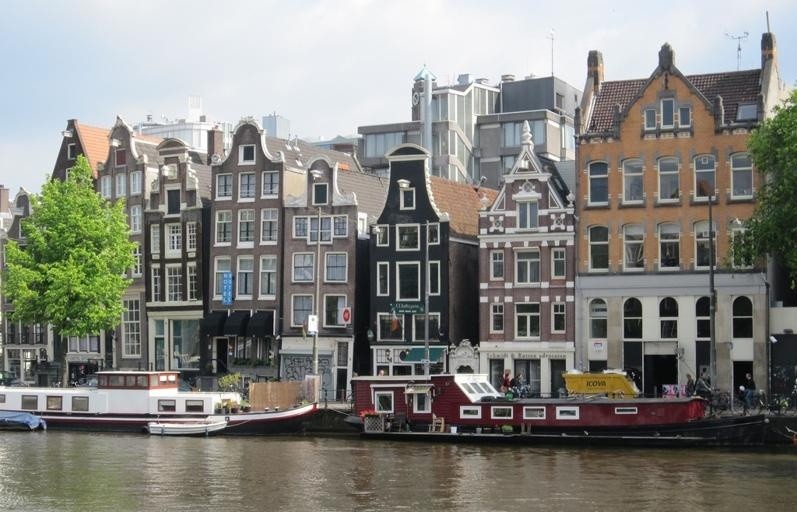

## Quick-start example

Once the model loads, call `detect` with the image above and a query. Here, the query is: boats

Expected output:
[145,418,229,436]
[344,372,773,451]
[0,411,47,432]
[0,369,320,420]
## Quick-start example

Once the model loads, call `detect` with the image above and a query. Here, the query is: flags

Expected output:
[392,311,398,332]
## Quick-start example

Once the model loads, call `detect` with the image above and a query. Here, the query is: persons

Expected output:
[739,373,756,408]
[685,368,714,402]
[632,370,640,390]
[503,372,531,399]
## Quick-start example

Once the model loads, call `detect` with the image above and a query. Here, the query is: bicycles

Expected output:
[746,392,788,418]
[693,390,731,410]
